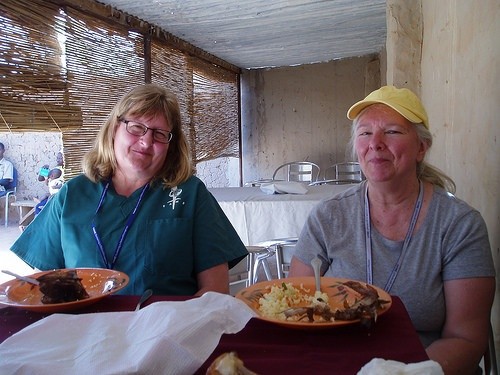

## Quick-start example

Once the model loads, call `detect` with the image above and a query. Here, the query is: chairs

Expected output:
[323,161,367,185]
[0,167,19,229]
[272,161,321,186]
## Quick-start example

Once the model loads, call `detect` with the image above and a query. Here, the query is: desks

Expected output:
[208,183,358,277]
[12,200,38,224]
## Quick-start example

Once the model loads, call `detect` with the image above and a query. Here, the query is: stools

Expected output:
[244,240,286,288]
[269,237,298,279]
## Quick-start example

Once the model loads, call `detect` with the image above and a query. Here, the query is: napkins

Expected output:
[0,291,258,375]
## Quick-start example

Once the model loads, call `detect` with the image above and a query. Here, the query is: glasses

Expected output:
[119,118,173,144]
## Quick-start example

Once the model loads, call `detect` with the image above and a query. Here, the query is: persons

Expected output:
[0,142,13,197]
[34,149,65,218]
[287,86,496,375]
[10,85,249,298]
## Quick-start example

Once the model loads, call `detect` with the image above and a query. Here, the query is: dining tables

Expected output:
[0,295,428,375]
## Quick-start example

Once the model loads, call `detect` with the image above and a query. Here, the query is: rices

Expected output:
[257,283,317,321]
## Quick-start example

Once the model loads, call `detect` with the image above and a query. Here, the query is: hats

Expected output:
[346,85,429,129]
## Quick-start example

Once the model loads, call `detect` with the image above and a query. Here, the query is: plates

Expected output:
[0,266,129,313]
[234,277,392,330]
